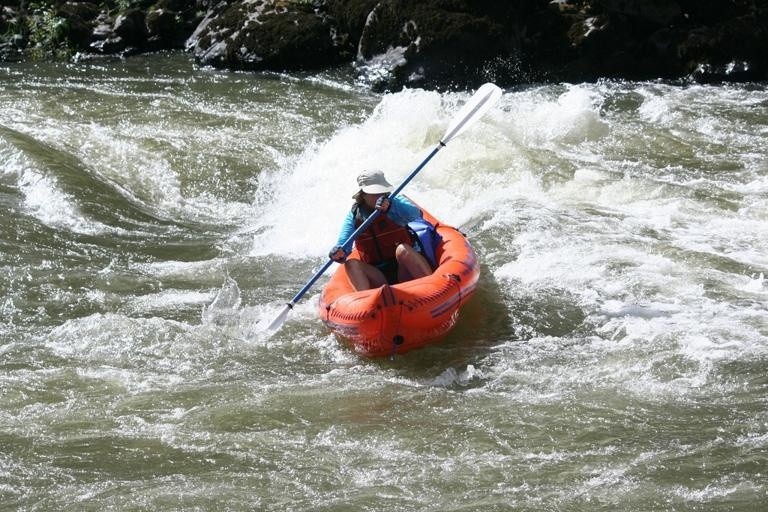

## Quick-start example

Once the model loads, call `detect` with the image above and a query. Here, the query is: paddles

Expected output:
[256,83,502,335]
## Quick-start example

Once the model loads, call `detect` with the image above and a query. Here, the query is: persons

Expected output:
[329,169,433,292]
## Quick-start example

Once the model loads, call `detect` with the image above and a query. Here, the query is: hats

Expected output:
[351,168,396,199]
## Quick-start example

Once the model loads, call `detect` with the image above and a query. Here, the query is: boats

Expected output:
[317,194,482,360]
